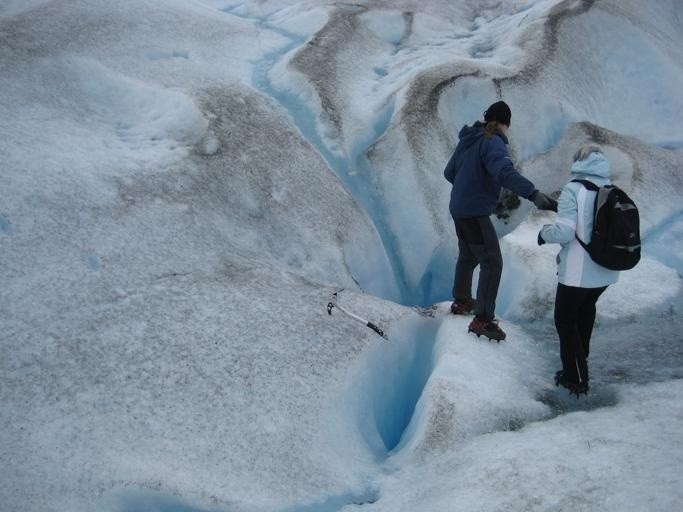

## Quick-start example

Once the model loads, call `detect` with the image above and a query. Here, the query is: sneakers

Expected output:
[555,370,590,396]
[467,318,506,343]
[450,298,477,315]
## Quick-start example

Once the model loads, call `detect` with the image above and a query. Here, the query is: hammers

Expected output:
[328,288,388,340]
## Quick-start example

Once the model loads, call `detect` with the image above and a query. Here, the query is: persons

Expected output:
[537,144,619,397]
[443,100,556,340]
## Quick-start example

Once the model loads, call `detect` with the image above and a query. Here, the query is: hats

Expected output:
[483,101,511,126]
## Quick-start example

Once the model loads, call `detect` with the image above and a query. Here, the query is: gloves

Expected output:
[534,192,557,212]
[538,231,545,246]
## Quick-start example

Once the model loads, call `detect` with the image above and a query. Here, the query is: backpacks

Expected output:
[569,179,642,271]
